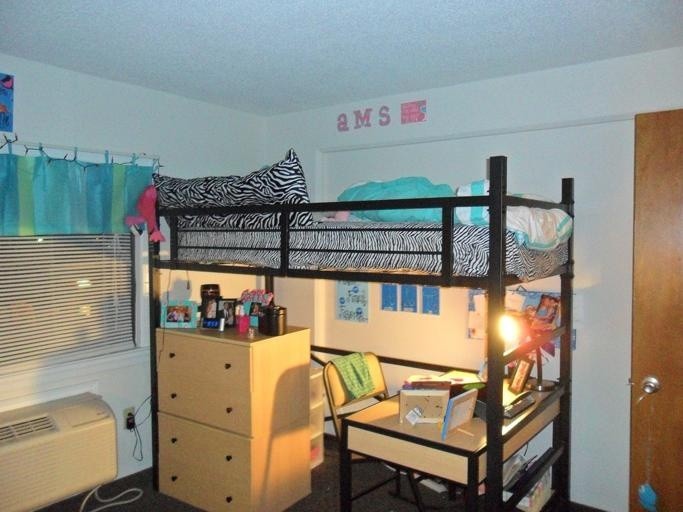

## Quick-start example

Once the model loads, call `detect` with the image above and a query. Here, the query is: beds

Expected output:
[151,156,574,512]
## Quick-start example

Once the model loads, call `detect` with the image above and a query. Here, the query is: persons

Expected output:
[536,296,550,324]
[538,298,557,323]
[166,298,235,325]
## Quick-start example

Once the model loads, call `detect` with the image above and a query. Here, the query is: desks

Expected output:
[340,370,561,512]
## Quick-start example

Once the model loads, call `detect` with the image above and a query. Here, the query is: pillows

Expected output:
[152,147,323,229]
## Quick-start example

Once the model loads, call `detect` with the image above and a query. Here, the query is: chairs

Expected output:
[323,352,428,512]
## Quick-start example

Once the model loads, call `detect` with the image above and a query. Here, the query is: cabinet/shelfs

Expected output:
[156,321,312,512]
[310,368,325,470]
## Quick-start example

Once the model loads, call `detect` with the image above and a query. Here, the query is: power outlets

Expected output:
[123,406,135,429]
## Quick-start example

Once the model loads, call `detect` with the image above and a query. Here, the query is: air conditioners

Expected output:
[0,392,118,512]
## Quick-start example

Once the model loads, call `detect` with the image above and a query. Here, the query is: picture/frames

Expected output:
[200,295,223,328]
[159,300,198,328]
[243,293,272,327]
[508,357,535,396]
[215,298,237,328]
[399,381,478,441]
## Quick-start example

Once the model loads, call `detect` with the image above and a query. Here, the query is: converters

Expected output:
[127,417,134,429]
